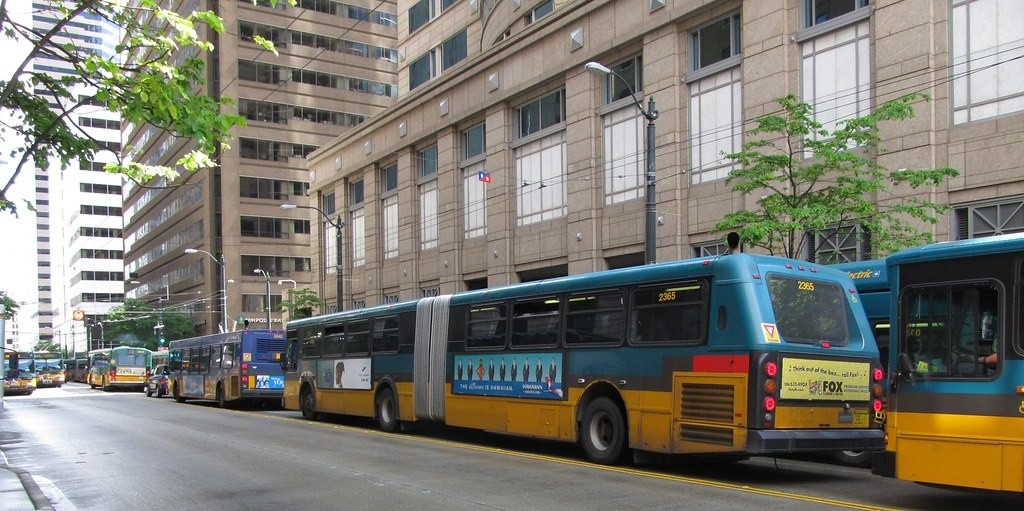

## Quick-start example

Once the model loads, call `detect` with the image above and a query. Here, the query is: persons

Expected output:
[457,357,558,382]
[974,353,999,364]
[336,362,346,387]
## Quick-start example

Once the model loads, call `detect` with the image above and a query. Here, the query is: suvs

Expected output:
[144,364,169,397]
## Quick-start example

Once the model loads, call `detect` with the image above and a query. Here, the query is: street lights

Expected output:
[90,325,94,352]
[255,269,271,329]
[131,280,164,347]
[185,248,227,332]
[586,61,657,264]
[280,204,344,313]
[278,280,297,315]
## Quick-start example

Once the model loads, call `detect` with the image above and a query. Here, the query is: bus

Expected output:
[4,352,37,394]
[887,231,1023,496]
[164,321,285,407]
[63,359,88,382]
[281,230,884,469]
[86,345,151,391]
[34,350,65,388]
[822,258,990,464]
[128,350,169,374]
